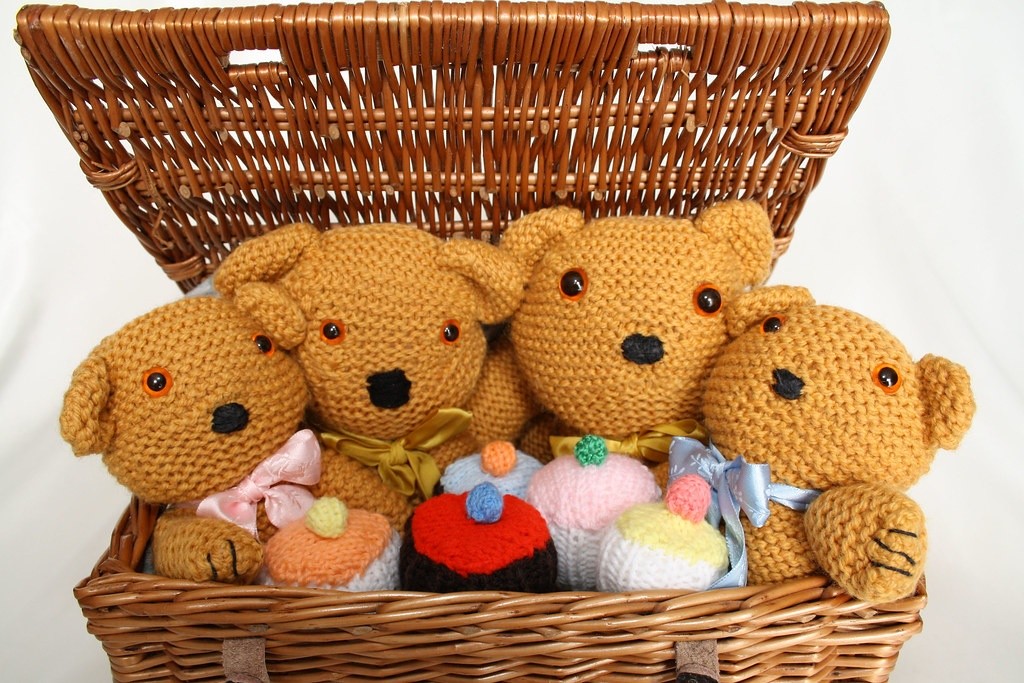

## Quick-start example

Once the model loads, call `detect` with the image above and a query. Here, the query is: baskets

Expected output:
[14,0,926,683]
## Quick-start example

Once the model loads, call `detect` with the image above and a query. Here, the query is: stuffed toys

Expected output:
[60,199,980,606]
